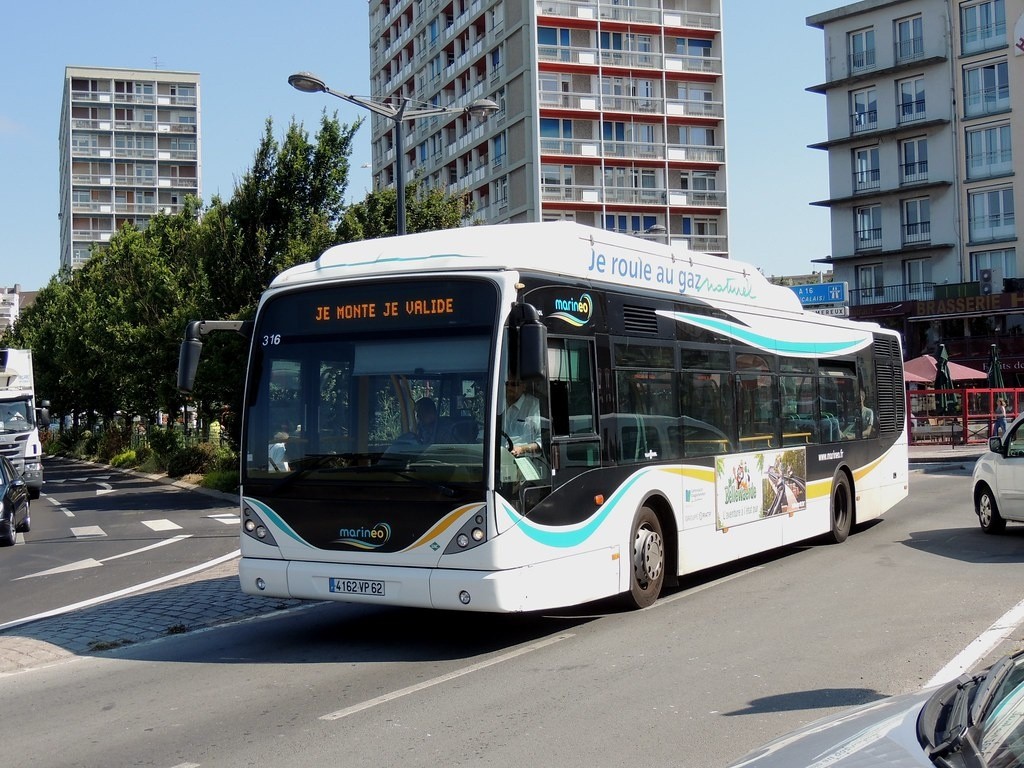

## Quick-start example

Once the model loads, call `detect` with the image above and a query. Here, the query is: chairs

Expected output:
[513,372,549,407]
[451,415,479,444]
[774,410,842,448]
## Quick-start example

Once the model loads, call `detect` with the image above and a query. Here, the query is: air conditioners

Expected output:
[979,267,1003,296]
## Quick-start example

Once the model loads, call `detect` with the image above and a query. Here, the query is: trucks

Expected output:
[0,348,53,500]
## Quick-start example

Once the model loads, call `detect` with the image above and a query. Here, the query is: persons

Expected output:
[267,432,288,470]
[993,398,1007,436]
[413,398,453,444]
[0,405,25,422]
[477,370,547,481]
[841,389,874,440]
[911,409,916,422]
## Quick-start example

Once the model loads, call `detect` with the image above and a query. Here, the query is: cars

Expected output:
[0,455,30,547]
[725,647,1024,768]
[971,412,1024,536]
[524,413,735,466]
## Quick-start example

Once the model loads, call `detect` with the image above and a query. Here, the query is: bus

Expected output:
[175,217,911,614]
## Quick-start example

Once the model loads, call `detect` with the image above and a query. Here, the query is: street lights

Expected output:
[288,70,501,238]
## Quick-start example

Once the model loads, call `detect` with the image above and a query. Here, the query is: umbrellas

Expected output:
[934,344,958,425]
[988,345,1007,404]
[904,354,987,418]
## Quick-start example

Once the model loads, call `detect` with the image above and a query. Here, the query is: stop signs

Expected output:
[730,353,768,392]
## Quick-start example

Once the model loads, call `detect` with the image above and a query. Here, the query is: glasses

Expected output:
[506,381,519,387]
